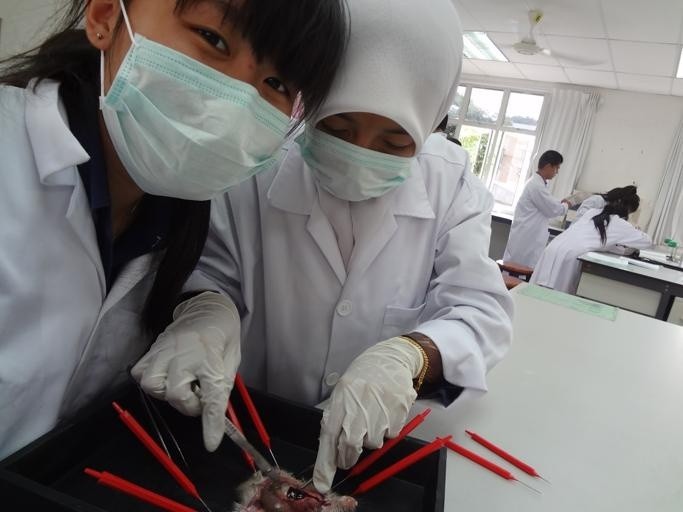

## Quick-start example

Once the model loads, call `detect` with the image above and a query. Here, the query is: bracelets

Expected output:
[402,334,430,394]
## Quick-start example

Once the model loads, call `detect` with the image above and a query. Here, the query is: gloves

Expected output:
[130,288,244,454]
[311,335,426,497]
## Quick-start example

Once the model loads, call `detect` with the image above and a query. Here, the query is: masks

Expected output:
[94,1,305,202]
[299,127,420,207]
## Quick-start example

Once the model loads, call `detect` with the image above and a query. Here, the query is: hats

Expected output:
[307,2,465,159]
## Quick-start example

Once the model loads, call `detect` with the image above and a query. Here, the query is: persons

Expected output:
[431,116,461,146]
[503,149,582,280]
[571,187,623,227]
[527,185,653,296]
[131,1,517,491]
[0,2,344,460]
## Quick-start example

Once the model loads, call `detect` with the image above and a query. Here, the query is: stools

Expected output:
[504,277,524,288]
[497,259,533,282]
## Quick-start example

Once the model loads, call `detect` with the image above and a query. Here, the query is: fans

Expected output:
[488,9,600,68]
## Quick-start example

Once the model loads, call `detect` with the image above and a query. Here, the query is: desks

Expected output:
[319,281,683,512]
[491,216,683,323]
